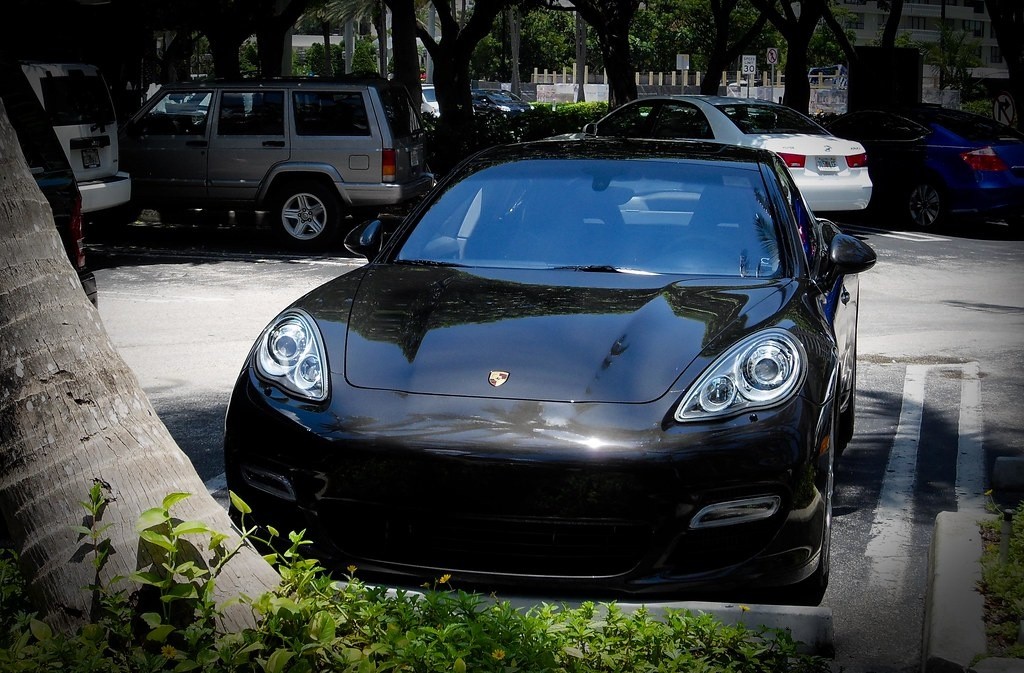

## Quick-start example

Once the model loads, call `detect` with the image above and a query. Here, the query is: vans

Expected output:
[20,63,132,214]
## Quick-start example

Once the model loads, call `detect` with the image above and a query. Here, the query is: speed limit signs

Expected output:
[742,54,756,74]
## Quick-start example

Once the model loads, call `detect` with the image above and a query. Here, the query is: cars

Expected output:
[471,88,536,120]
[0,60,98,295]
[420,82,441,118]
[541,94,873,212]
[224,131,880,606]
[114,74,438,256]
[823,104,1024,234]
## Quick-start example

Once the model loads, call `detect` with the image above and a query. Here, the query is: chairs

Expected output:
[757,111,776,129]
[658,110,691,137]
[687,181,770,267]
[556,185,629,253]
